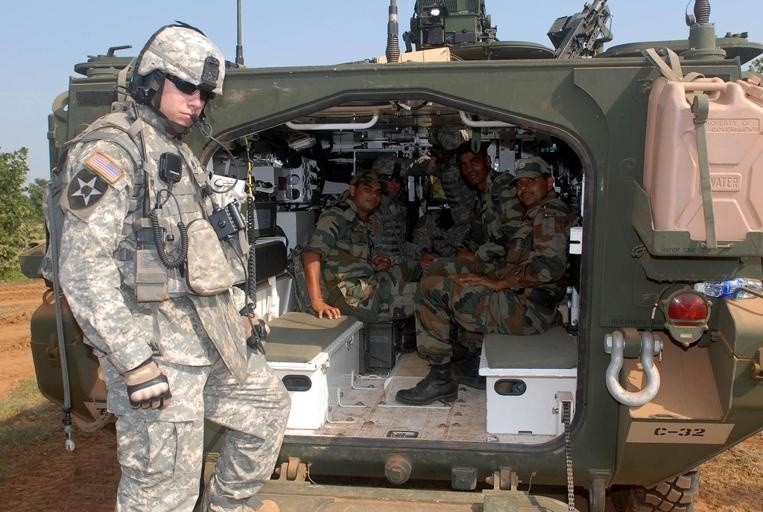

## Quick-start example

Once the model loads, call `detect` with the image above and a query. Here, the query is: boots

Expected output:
[396,362,458,405]
[396,316,416,353]
[453,344,486,390]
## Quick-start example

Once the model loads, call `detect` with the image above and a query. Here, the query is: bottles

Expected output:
[693,277,763,300]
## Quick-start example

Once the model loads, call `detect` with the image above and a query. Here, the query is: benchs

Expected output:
[262,310,363,430]
[478,325,578,435]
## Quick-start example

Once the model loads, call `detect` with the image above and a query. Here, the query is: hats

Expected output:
[508,156,552,187]
[350,169,387,192]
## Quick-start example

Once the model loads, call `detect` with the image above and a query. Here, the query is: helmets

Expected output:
[137,26,225,96]
[373,154,408,177]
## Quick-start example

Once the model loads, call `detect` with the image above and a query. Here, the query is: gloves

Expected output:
[120,357,171,410]
[241,312,271,348]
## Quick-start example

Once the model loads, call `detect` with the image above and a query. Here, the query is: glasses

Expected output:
[378,174,404,183]
[163,73,215,101]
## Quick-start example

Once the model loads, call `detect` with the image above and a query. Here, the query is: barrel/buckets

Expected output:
[643,75,763,241]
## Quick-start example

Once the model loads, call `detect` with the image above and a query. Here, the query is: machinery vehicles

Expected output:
[19,1,762,511]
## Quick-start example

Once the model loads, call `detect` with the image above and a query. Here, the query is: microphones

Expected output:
[188,110,212,138]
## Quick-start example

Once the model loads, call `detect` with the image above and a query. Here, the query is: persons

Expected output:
[49,22,292,512]
[449,139,525,280]
[367,154,436,291]
[297,169,416,323]
[417,123,483,256]
[393,155,581,405]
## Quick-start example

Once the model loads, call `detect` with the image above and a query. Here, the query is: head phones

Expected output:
[130,24,164,104]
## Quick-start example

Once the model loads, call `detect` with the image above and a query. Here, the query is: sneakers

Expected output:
[208,499,279,512]
